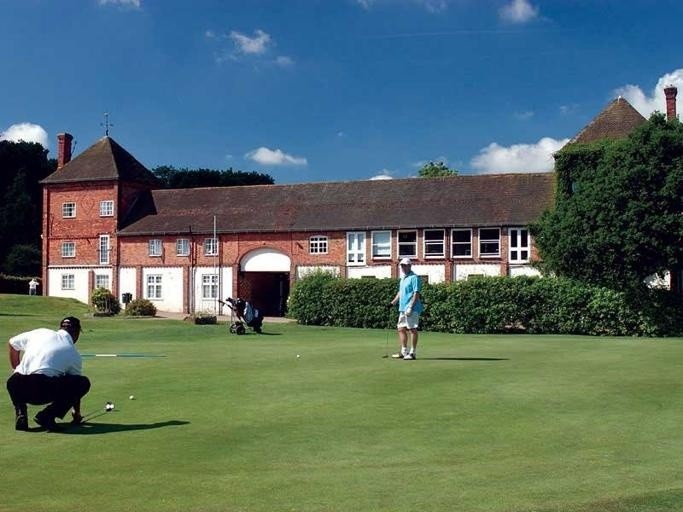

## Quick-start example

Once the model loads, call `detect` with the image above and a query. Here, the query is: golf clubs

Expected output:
[80,401,112,419]
[381,307,390,359]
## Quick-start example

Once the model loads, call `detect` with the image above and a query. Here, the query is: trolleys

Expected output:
[220,297,265,336]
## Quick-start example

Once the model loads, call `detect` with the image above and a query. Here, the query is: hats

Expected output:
[399,258,411,265]
[60,318,80,329]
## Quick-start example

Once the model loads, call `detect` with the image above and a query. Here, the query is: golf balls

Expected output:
[297,355,299,357]
[105,395,134,410]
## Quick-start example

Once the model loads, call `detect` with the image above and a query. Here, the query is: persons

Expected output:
[27,277,39,296]
[386,258,423,359]
[7,316,90,431]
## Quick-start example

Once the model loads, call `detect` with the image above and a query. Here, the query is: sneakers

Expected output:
[15,409,28,430]
[392,353,416,360]
[33,411,56,429]
